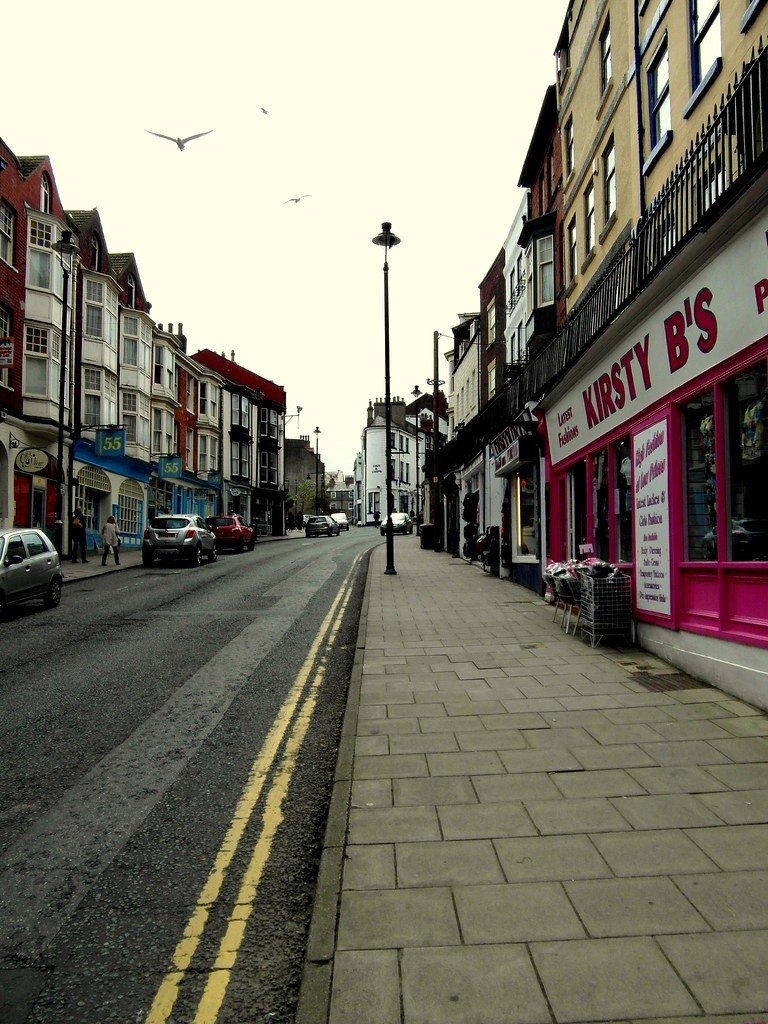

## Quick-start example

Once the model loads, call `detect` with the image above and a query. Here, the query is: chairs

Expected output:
[553,576,583,635]
[544,571,566,629]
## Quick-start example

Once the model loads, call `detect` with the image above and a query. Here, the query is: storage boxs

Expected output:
[577,568,633,648]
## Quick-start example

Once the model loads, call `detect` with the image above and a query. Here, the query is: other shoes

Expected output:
[71,560,79,564]
[116,562,121,565]
[102,562,106,566]
[82,560,90,563]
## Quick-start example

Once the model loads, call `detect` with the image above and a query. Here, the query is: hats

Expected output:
[107,516,115,523]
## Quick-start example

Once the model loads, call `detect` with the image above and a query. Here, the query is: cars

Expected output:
[305,515,340,537]
[379,512,413,535]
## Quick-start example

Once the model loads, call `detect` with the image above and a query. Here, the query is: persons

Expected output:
[101,516,121,566]
[373,512,380,528]
[71,508,90,563]
[410,509,415,522]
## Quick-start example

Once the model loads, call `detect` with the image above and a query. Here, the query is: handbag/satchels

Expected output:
[117,536,122,546]
[72,518,83,528]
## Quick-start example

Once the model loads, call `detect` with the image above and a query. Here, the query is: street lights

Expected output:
[371,222,402,578]
[50,229,79,560]
[313,426,322,517]
[410,384,420,535]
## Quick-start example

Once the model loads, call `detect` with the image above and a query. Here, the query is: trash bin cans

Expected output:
[419,522,435,550]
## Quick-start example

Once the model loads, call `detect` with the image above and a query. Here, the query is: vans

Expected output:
[331,512,350,531]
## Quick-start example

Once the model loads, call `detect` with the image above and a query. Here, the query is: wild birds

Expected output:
[144,129,214,152]
[284,195,311,203]
[261,108,268,114]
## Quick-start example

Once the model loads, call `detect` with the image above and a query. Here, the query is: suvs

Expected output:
[203,512,255,551]
[143,513,219,564]
[0,526,64,613]
